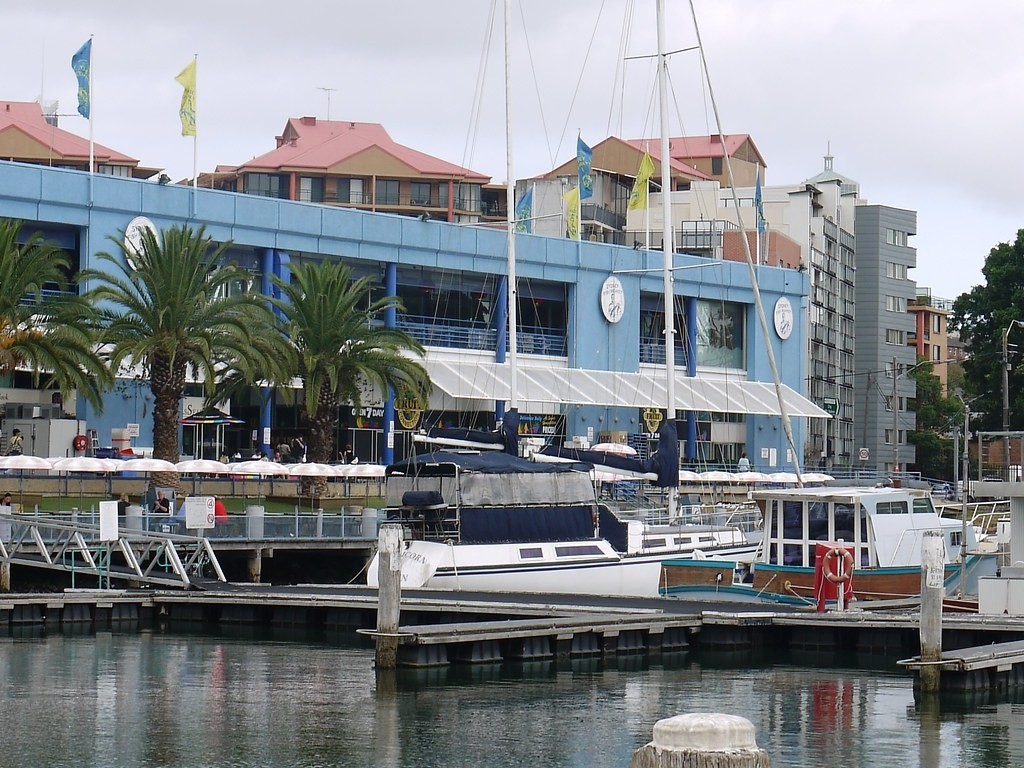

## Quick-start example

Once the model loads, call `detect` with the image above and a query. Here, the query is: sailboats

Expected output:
[363,0,1024,592]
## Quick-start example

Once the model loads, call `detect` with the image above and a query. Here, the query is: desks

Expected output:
[11,512,388,540]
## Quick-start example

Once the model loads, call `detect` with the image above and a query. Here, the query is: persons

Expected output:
[150,491,170,531]
[818,457,833,474]
[273,434,306,462]
[176,494,226,538]
[0,492,12,506]
[117,493,131,527]
[4,428,23,456]
[253,449,262,458]
[220,450,241,464]
[339,445,354,464]
[737,453,751,472]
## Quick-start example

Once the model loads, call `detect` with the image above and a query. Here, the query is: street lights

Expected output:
[1002,320,1024,483]
[893,355,955,475]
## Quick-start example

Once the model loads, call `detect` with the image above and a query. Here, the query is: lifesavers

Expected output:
[822,547,854,583]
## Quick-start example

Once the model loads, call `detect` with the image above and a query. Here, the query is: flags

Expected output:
[755,172,766,235]
[71,38,91,120]
[516,189,533,233]
[577,137,592,199]
[628,152,655,210]
[562,188,578,239]
[175,60,196,136]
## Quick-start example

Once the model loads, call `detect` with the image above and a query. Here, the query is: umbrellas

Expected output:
[592,442,637,454]
[680,470,835,501]
[0,455,404,513]
[178,406,244,460]
